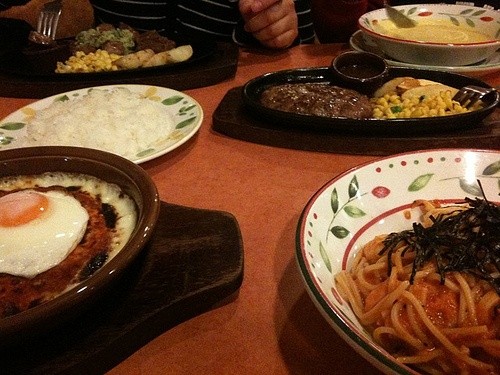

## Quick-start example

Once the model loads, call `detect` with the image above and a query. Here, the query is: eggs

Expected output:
[0,188,90,276]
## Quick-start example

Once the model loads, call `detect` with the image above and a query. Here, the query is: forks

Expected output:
[37,0,63,41]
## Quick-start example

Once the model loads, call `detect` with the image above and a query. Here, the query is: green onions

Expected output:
[76,26,135,54]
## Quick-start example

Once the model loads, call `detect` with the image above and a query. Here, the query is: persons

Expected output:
[0,0,317,49]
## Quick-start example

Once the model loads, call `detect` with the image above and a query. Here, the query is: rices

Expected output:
[8,88,174,156]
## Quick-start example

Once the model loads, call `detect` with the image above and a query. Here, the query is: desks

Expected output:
[0,44,500,375]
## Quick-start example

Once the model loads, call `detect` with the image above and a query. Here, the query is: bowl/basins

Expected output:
[357,3,500,65]
[0,145,160,332]
[289,148,500,375]
[332,51,389,92]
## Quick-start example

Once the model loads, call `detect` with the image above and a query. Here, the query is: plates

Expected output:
[0,31,219,80]
[242,65,500,133]
[0,83,204,164]
[350,28,500,78]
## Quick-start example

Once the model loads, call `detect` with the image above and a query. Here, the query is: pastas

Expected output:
[335,200,500,375]
